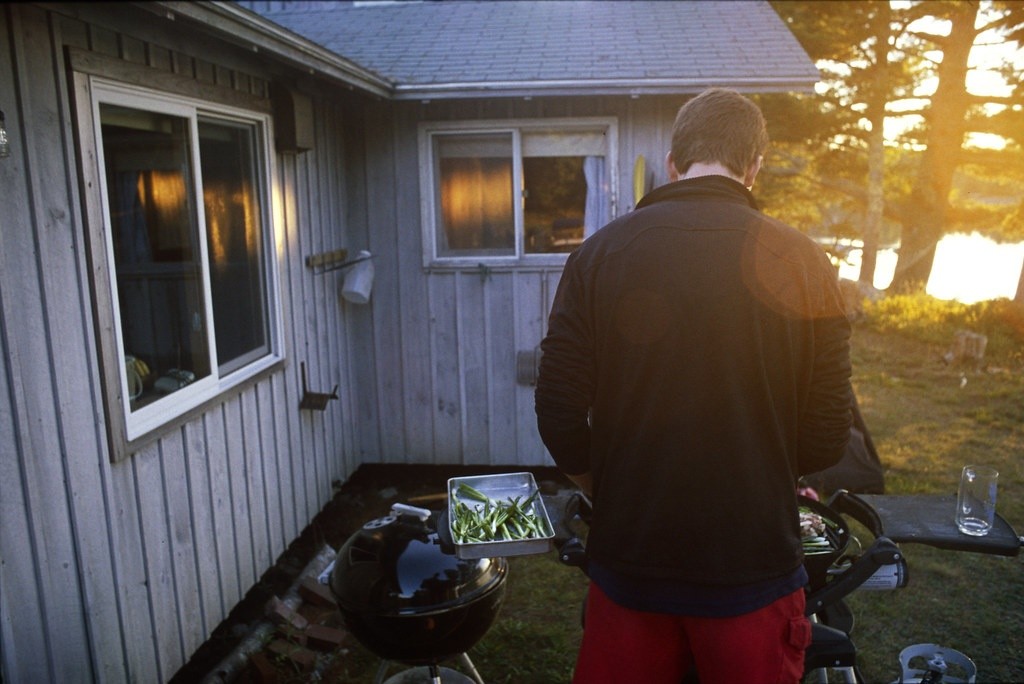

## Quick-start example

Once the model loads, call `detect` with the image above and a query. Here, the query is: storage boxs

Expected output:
[448,472,555,560]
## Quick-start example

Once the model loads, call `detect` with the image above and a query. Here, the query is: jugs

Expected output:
[342,250,375,303]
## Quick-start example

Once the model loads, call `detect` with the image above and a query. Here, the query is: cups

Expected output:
[955,464,999,537]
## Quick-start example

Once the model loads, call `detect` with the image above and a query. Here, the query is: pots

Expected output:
[327,506,508,668]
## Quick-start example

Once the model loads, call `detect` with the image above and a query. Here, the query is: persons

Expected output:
[534,89,853,684]
